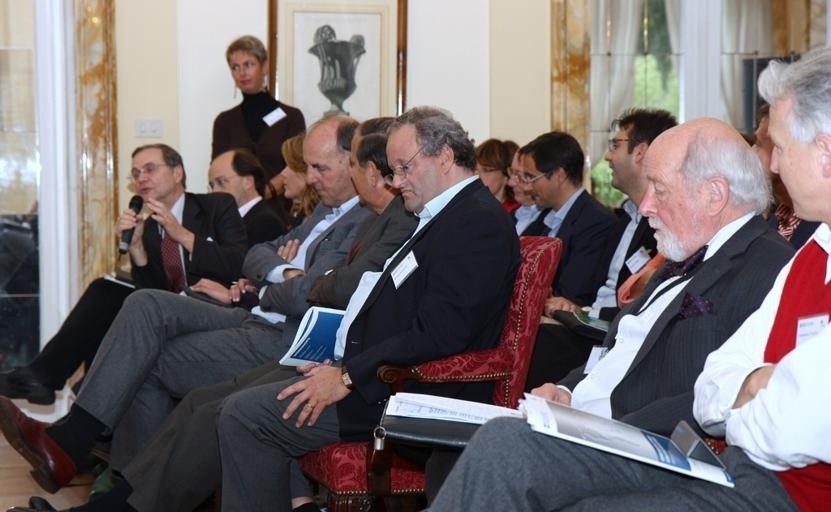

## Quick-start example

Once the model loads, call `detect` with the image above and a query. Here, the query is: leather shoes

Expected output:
[1,396,77,494]
[0,367,55,405]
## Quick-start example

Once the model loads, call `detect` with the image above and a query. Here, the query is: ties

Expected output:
[161,228,186,294]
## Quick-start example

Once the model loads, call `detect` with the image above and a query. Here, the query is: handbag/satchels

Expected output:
[379,400,481,447]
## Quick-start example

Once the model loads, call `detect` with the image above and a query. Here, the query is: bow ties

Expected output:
[660,245,708,280]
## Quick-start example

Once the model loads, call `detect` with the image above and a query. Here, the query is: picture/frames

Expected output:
[266,0,408,132]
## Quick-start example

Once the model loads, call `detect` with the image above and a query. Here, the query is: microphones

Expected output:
[118,193,145,256]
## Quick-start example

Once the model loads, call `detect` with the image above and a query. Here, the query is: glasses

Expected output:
[608,137,630,151]
[474,168,498,173]
[507,167,520,179]
[132,164,166,179]
[521,173,544,184]
[384,146,425,187]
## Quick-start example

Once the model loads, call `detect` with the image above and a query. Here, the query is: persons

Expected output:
[0,35,829,511]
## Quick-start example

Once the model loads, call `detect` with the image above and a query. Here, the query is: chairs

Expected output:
[295,236,564,512]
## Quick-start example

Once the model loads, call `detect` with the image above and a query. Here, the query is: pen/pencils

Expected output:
[231,281,238,285]
